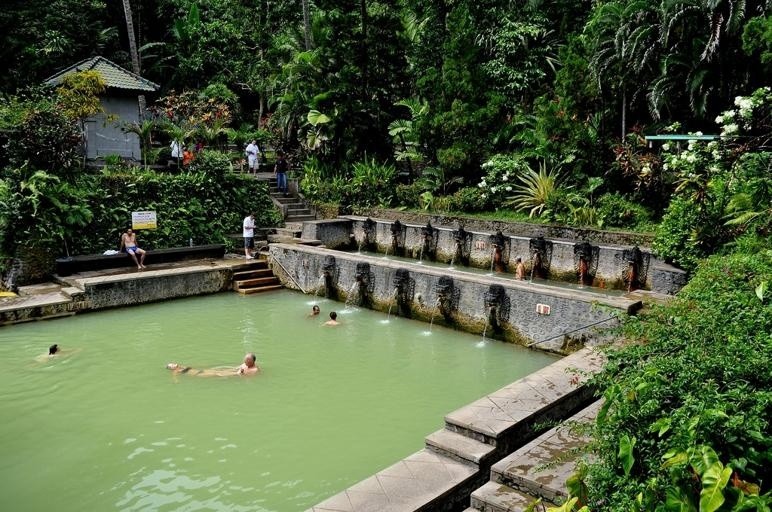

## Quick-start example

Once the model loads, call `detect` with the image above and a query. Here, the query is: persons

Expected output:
[50,344,60,355]
[170,141,194,168]
[246,139,261,177]
[273,152,289,195]
[515,257,526,280]
[119,228,146,269]
[167,353,260,377]
[243,212,257,260]
[326,311,340,325]
[310,305,320,316]
